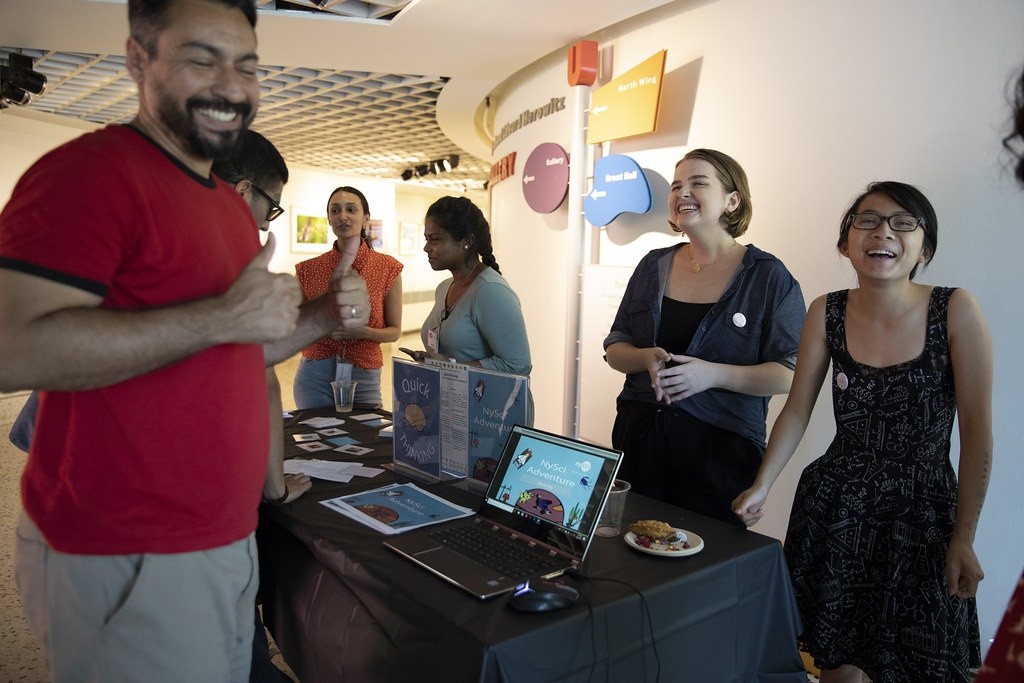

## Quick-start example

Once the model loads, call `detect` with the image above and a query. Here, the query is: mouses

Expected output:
[506,577,580,613]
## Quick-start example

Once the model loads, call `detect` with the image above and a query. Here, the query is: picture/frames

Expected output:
[290,205,332,253]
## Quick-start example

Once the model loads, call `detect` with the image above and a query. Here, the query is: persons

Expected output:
[602,148,806,532]
[399,195,535,427]
[7,132,312,683]
[0,1,376,683]
[735,181,993,683]
[292,186,403,408]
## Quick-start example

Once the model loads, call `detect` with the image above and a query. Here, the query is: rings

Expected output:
[351,305,355,318]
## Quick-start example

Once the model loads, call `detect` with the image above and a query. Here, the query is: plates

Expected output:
[624,527,704,556]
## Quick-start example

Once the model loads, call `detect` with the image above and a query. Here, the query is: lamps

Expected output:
[401,155,459,181]
[0,51,47,109]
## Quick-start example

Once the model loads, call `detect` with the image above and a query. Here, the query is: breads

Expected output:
[628,520,676,537]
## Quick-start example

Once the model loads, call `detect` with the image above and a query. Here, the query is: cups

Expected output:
[594,479,630,537]
[330,380,358,412]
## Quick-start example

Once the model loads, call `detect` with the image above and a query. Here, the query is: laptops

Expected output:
[382,423,624,598]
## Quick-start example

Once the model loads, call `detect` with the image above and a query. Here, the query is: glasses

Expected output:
[845,212,924,232]
[233,178,284,222]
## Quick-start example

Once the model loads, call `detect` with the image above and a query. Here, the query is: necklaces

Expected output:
[440,261,481,320]
[687,242,736,273]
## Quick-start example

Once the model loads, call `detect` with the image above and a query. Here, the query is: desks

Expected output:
[250,405,810,683]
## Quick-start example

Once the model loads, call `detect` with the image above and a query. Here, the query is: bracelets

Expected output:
[263,484,289,503]
[449,357,456,363]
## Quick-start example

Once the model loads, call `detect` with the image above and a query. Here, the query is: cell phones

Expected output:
[399,347,421,361]
